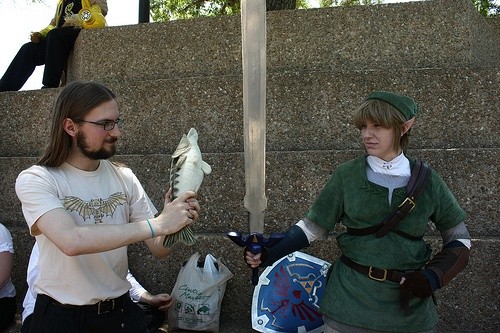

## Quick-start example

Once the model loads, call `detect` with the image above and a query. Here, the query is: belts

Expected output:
[339,253,409,285]
[36,290,132,315]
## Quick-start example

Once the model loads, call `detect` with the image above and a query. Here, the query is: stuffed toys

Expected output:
[161,127,212,248]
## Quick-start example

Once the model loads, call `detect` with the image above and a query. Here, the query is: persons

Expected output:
[21,240,175,332]
[0,0,108,92]
[242,91,472,333]
[15,82,201,333]
[0,223,15,333]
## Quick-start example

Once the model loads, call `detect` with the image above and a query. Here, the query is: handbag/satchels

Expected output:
[166,252,234,333]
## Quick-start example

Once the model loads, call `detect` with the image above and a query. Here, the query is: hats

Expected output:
[364,90,418,136]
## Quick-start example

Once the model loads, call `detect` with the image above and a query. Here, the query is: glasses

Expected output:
[73,118,119,131]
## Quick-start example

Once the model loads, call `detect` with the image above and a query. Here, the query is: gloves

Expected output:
[403,239,469,298]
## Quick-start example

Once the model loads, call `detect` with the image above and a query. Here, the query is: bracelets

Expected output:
[146,219,155,239]
